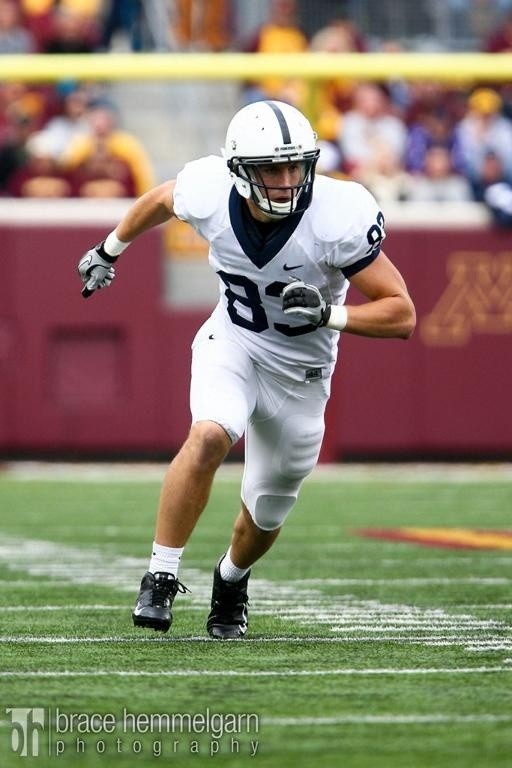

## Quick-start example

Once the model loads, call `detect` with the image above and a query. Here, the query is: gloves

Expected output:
[280,279,333,331]
[74,238,117,297]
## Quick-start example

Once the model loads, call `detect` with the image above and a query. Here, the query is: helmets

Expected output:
[222,100,321,199]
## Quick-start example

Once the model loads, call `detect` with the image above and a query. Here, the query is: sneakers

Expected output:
[131,569,178,633]
[204,551,250,640]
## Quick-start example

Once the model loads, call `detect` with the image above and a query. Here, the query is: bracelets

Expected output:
[326,304,348,333]
[104,231,131,259]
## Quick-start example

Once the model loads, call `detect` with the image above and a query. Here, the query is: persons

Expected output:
[80,99,418,639]
[0,1,512,233]
